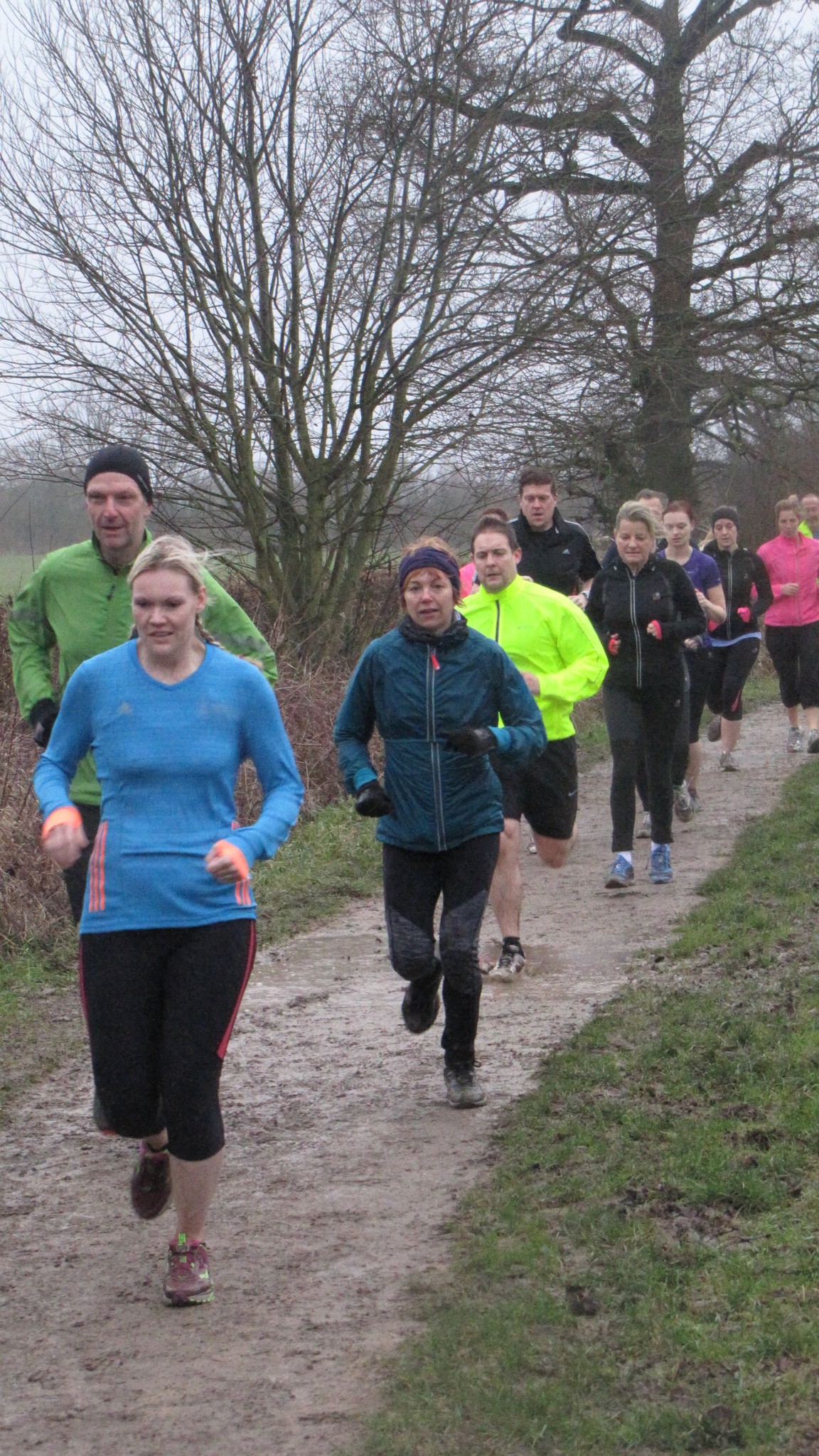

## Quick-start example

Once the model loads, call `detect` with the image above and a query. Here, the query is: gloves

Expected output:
[737,606,750,624]
[29,698,58,748]
[444,725,496,761]
[354,779,397,820]
[707,619,717,634]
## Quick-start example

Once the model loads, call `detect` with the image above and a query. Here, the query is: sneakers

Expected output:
[402,956,443,1034]
[708,713,725,742]
[442,1055,487,1110]
[131,1140,172,1223]
[671,782,696,825]
[605,854,637,892]
[489,948,528,984]
[635,811,654,841]
[807,729,819,754]
[644,845,675,885]
[162,1232,217,1307]
[784,725,805,754]
[686,786,701,811]
[719,750,740,773]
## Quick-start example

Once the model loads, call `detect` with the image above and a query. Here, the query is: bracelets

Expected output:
[579,591,591,601]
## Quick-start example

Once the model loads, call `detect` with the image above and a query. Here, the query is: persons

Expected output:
[333,536,550,1110]
[5,441,278,937]
[450,508,512,603]
[602,488,818,837]
[32,534,312,1305]
[505,470,605,614]
[583,500,710,892]
[447,518,613,988]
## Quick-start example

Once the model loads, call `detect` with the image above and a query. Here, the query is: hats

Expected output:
[82,444,156,504]
[710,505,743,535]
